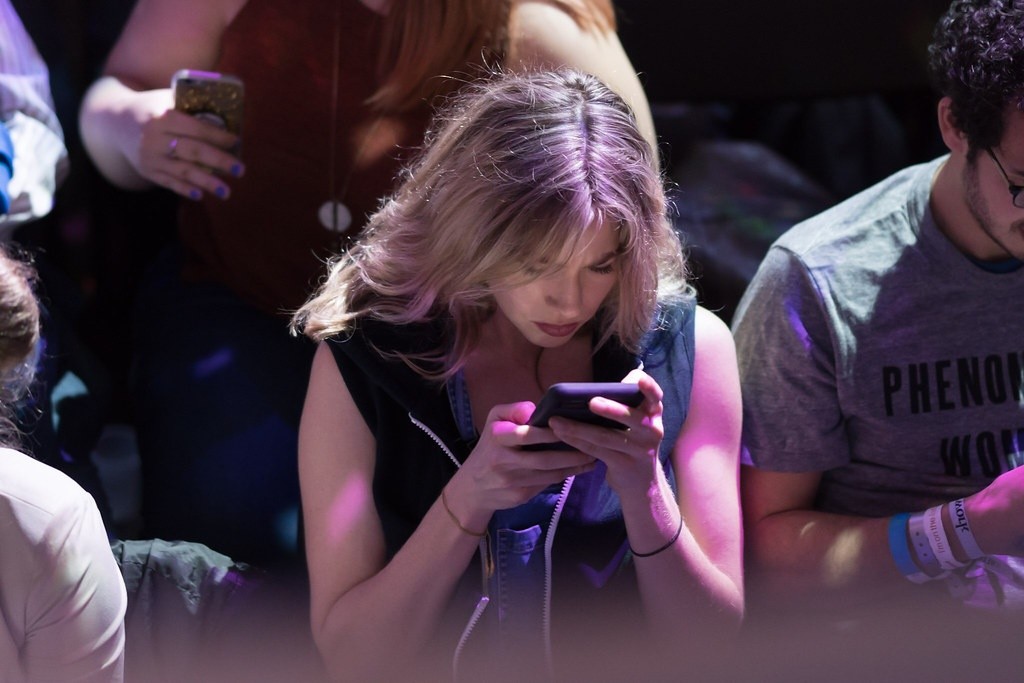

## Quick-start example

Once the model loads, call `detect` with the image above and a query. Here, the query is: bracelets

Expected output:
[441,488,486,537]
[628,513,683,558]
[889,498,1024,608]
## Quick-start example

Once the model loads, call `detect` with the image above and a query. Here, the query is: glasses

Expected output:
[983,145,1024,207]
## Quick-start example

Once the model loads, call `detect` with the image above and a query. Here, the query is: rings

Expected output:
[166,139,178,155]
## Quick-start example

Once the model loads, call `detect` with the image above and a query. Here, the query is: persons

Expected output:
[0,0,745,683]
[731,0,1024,683]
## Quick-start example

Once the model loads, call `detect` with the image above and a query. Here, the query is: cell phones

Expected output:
[172,70,245,136]
[518,381,645,453]
